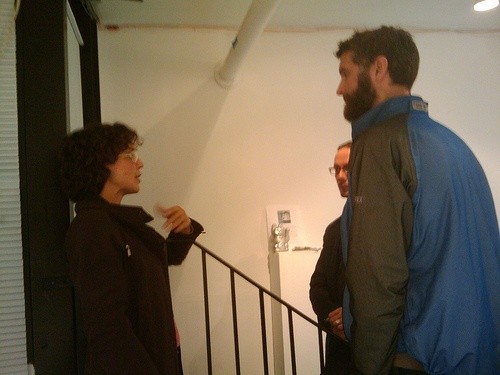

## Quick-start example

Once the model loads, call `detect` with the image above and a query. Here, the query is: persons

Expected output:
[61,124,203,375]
[334,26,500,375]
[309,141,353,374]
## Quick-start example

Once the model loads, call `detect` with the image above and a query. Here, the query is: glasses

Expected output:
[119,150,140,163]
[328,164,349,177]
